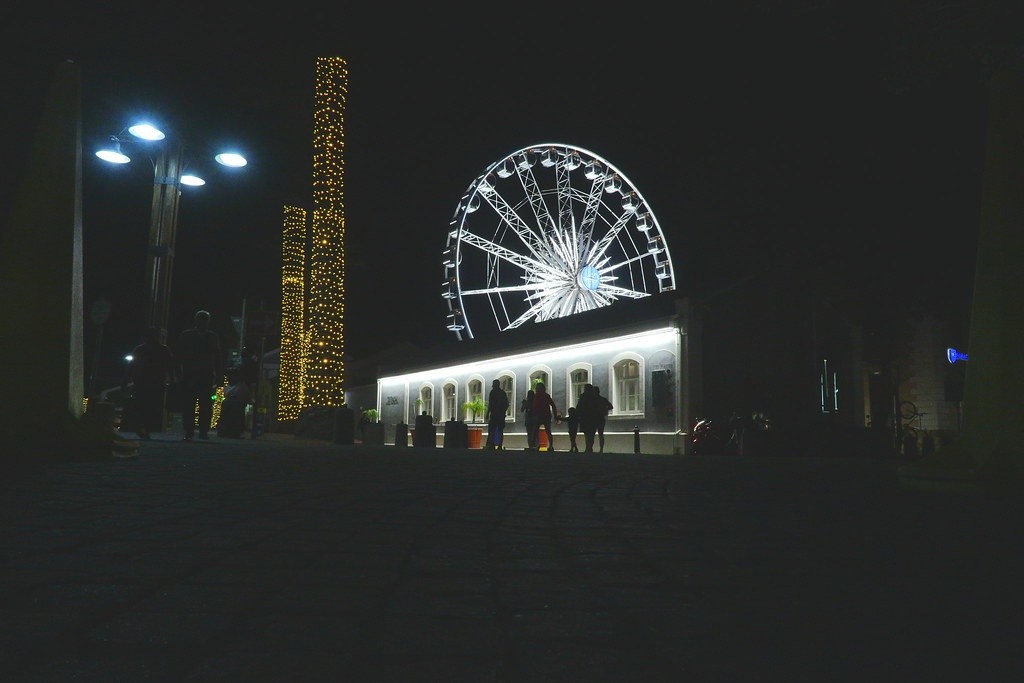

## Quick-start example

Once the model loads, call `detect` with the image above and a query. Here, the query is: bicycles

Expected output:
[901,400,930,448]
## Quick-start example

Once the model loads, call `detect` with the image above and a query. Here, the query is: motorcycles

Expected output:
[690,416,723,455]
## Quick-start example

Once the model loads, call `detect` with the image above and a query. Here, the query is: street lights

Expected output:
[93,108,250,435]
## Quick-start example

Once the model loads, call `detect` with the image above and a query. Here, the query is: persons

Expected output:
[120,310,222,442]
[485,380,509,450]
[521,382,613,456]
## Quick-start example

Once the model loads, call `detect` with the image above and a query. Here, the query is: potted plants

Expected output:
[410,397,424,445]
[460,392,488,448]
[360,409,384,445]
[531,378,547,448]
